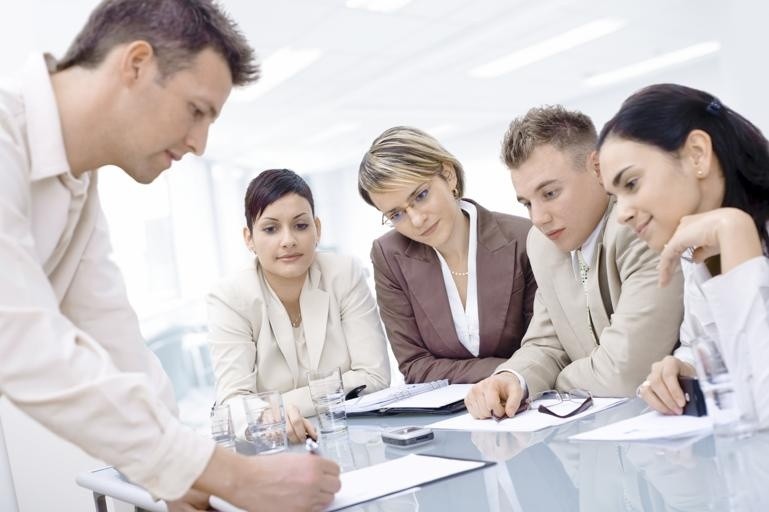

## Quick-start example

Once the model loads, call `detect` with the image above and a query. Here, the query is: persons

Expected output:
[0,1,343,512]
[593,82,769,421]
[463,104,684,421]
[357,126,539,387]
[202,167,394,446]
[314,424,768,511]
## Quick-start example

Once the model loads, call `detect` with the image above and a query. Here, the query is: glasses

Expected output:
[381,179,432,227]
[491,389,592,420]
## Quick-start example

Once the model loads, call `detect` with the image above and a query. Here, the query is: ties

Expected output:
[575,248,599,348]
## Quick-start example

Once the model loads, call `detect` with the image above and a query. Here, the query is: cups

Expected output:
[692,333,760,443]
[243,391,286,455]
[211,405,231,443]
[306,367,348,436]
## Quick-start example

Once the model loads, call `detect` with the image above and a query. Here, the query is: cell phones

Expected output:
[381,425,435,449]
[678,373,708,417]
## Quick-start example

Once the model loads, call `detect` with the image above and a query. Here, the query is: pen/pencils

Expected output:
[306,438,321,456]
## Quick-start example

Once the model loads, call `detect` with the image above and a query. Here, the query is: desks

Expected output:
[75,396,769,512]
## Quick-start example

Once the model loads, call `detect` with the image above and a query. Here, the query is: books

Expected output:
[332,377,476,416]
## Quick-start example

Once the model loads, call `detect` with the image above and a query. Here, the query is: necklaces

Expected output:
[291,313,301,326]
[451,271,468,276]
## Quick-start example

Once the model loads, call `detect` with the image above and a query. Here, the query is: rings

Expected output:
[641,380,651,387]
